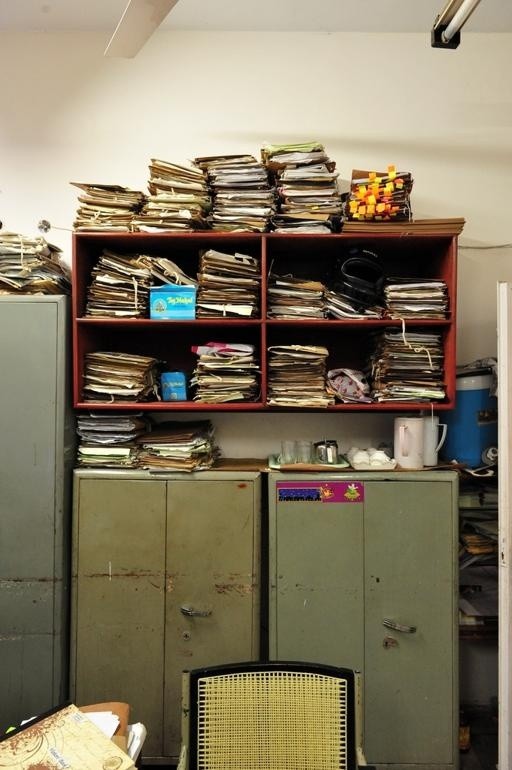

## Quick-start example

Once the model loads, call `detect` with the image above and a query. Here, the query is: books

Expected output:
[82,250,262,318]
[370,332,445,403]
[77,411,221,472]
[266,273,447,320]
[83,351,161,405]
[192,343,262,403]
[1,230,72,299]
[1,693,149,770]
[265,343,334,407]
[72,142,343,234]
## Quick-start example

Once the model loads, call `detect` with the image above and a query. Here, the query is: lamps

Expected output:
[430,0,482,52]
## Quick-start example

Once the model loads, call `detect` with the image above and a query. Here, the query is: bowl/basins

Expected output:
[347,446,392,465]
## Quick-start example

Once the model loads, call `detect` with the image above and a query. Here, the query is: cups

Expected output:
[393,416,426,469]
[276,437,339,465]
[417,414,448,468]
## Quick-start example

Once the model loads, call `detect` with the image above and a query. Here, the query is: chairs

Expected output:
[179,660,367,770]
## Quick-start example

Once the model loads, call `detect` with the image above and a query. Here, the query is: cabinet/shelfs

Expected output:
[66,216,466,417]
[67,459,264,767]
[263,459,460,770]
